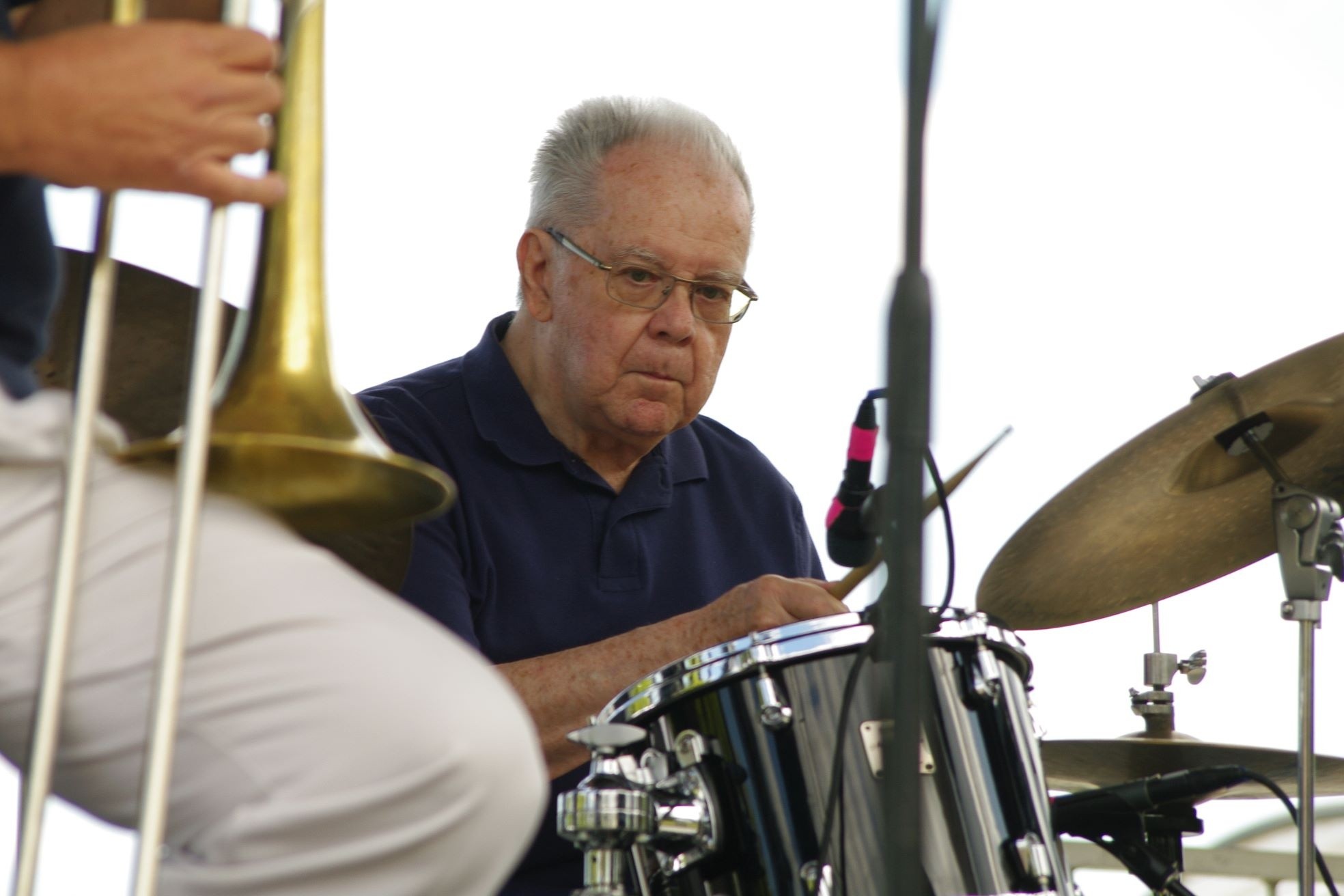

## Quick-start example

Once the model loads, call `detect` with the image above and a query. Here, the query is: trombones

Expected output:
[14,0,454,896]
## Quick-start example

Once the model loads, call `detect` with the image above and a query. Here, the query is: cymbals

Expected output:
[1038,728,1344,803]
[977,333,1343,630]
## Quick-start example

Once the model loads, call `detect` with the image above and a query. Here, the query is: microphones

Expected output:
[827,397,879,567]
[1052,760,1243,837]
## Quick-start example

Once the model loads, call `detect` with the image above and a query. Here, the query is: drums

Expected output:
[559,611,1076,896]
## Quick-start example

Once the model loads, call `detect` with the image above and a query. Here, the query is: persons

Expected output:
[355,92,850,896]
[0,0,556,896]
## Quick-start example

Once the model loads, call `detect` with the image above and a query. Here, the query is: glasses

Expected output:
[542,225,759,324]
